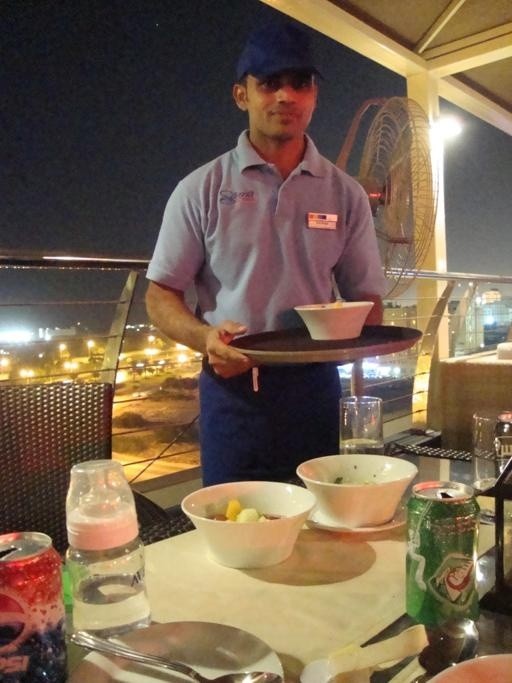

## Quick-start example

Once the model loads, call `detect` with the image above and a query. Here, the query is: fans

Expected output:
[351,96,442,395]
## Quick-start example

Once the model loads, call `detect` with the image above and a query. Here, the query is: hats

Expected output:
[238,24,322,83]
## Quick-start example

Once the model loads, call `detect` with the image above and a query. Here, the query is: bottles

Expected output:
[66,459,150,639]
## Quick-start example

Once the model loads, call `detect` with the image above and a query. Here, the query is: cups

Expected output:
[295,455,418,526]
[338,395,385,455]
[473,408,512,490]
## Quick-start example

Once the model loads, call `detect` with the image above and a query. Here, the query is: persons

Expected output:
[144,20,392,489]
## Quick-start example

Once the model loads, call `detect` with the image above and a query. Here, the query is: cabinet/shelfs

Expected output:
[413,271,512,453]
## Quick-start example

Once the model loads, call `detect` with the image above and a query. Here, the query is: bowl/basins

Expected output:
[180,481,315,571]
[295,300,374,341]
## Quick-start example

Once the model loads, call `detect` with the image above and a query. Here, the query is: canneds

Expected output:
[0,531,68,683]
[404,481,481,630]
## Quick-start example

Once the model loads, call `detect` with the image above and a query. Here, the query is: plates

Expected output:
[308,507,408,533]
[64,620,284,683]
[426,654,511,683]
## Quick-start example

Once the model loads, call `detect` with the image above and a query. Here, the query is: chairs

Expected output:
[0,381,195,559]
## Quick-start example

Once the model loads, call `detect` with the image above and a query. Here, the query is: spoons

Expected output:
[71,630,282,683]
[412,615,478,683]
